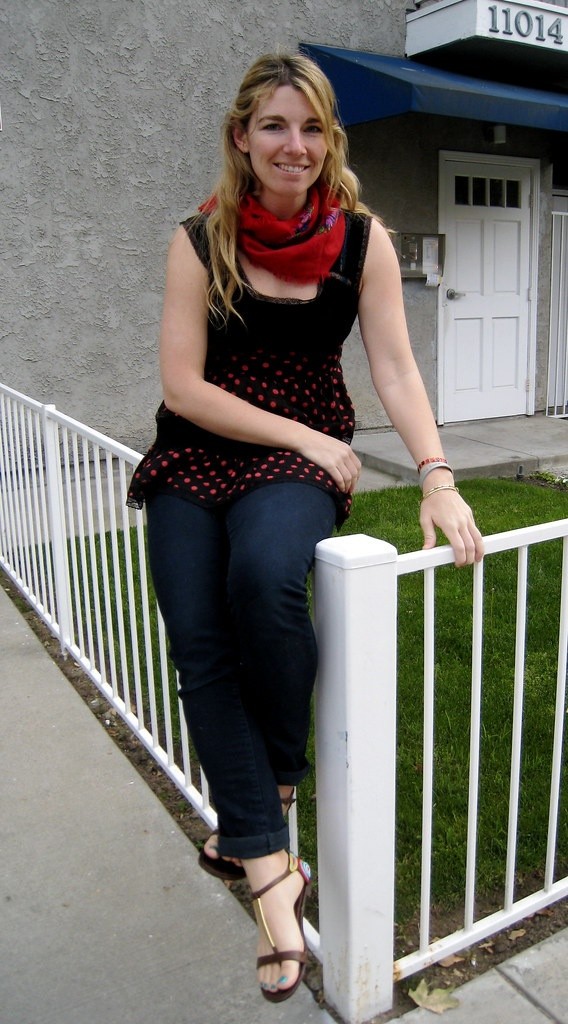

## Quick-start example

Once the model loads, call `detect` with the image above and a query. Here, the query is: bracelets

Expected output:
[423,486,460,497]
[418,457,447,473]
[419,462,454,490]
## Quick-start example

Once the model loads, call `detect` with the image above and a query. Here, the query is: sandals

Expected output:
[197,787,296,880]
[252,852,312,1003]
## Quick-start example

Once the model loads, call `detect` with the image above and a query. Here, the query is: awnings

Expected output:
[299,44,568,167]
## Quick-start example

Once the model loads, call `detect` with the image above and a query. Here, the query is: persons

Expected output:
[127,54,486,1002]
